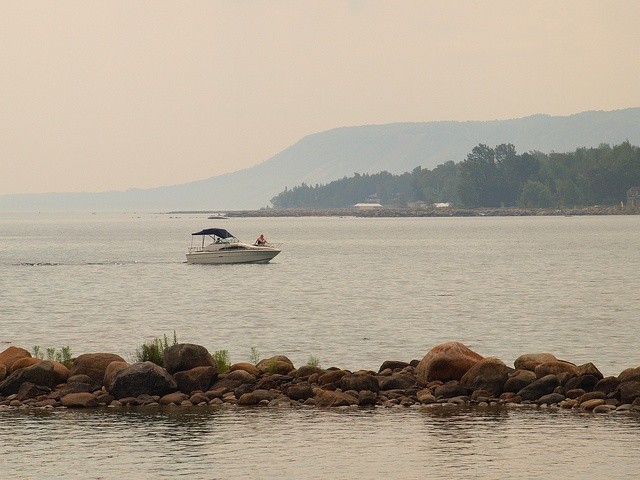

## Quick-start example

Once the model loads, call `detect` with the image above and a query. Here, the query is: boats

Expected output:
[185,228,284,265]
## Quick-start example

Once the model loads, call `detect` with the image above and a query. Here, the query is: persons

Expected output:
[254,234,270,245]
